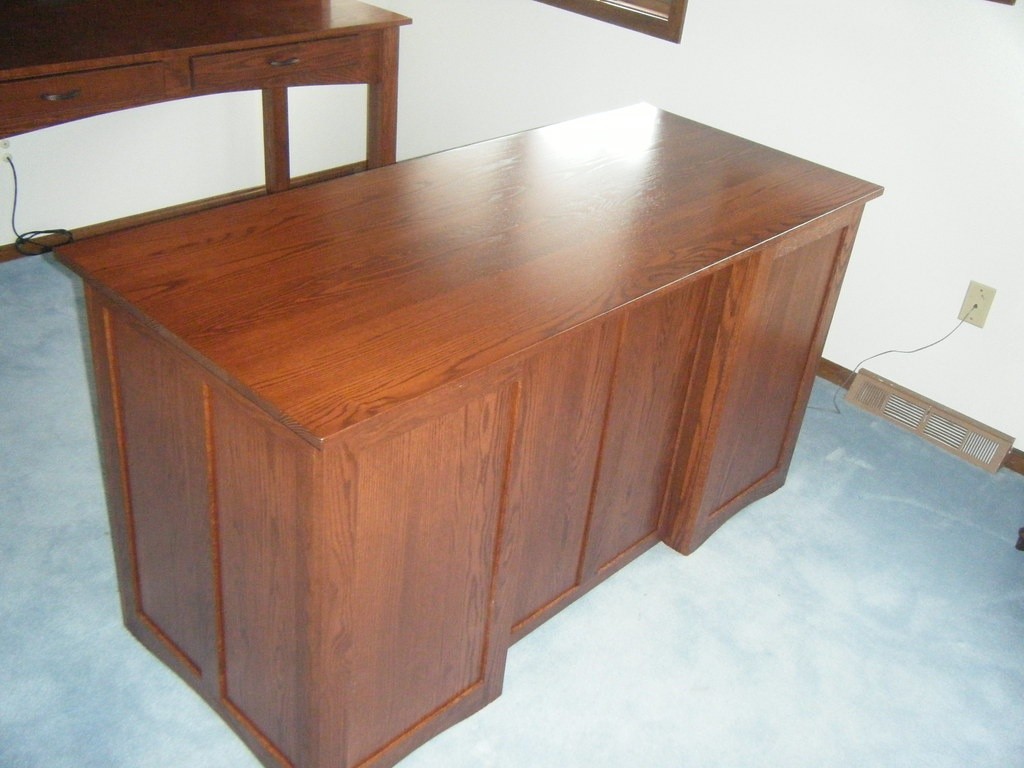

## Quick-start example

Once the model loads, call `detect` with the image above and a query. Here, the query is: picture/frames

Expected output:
[537,0,689,46]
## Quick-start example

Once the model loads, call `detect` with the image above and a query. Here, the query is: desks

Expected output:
[53,100,885,768]
[0,0,412,172]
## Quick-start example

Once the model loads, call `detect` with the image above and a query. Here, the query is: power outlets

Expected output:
[958,279,996,328]
[0,138,13,166]
